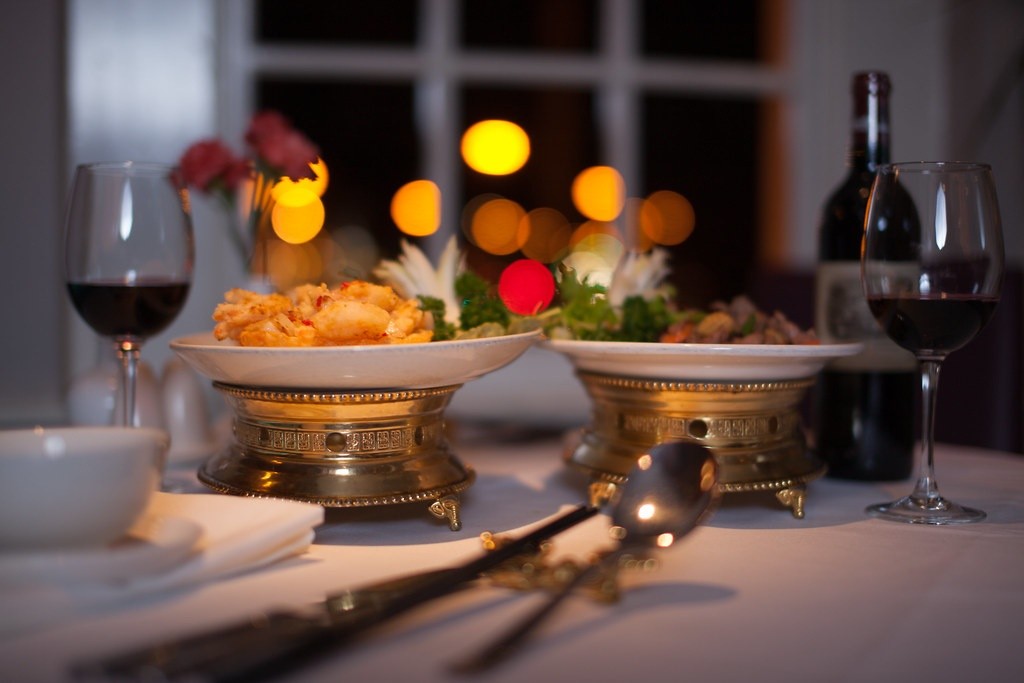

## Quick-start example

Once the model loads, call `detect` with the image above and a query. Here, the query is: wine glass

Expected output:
[63,163,195,493]
[860,161,1005,524]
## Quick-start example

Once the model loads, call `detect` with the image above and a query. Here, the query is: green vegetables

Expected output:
[416,268,754,345]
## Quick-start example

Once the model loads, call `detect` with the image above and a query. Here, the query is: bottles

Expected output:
[817,70,924,484]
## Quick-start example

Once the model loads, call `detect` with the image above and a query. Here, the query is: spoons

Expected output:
[445,439,720,683]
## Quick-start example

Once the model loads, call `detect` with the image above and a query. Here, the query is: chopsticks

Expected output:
[72,502,600,683]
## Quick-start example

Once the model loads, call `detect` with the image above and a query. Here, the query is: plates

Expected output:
[170,328,542,387]
[545,328,862,381]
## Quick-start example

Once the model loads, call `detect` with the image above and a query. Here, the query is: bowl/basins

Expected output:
[0,428,174,549]
